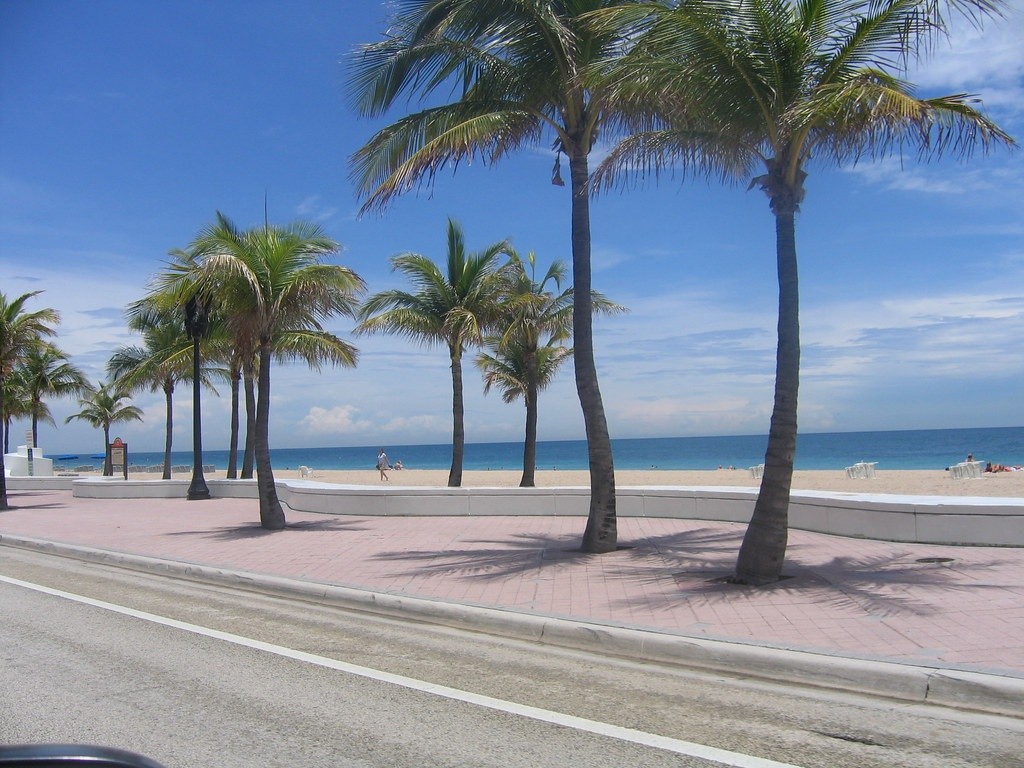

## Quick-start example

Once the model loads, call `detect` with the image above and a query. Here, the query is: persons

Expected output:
[377,447,392,481]
[965,453,974,462]
[986,462,1022,473]
[396,460,401,469]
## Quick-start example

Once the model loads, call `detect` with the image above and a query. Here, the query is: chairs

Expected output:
[301,466,313,479]
